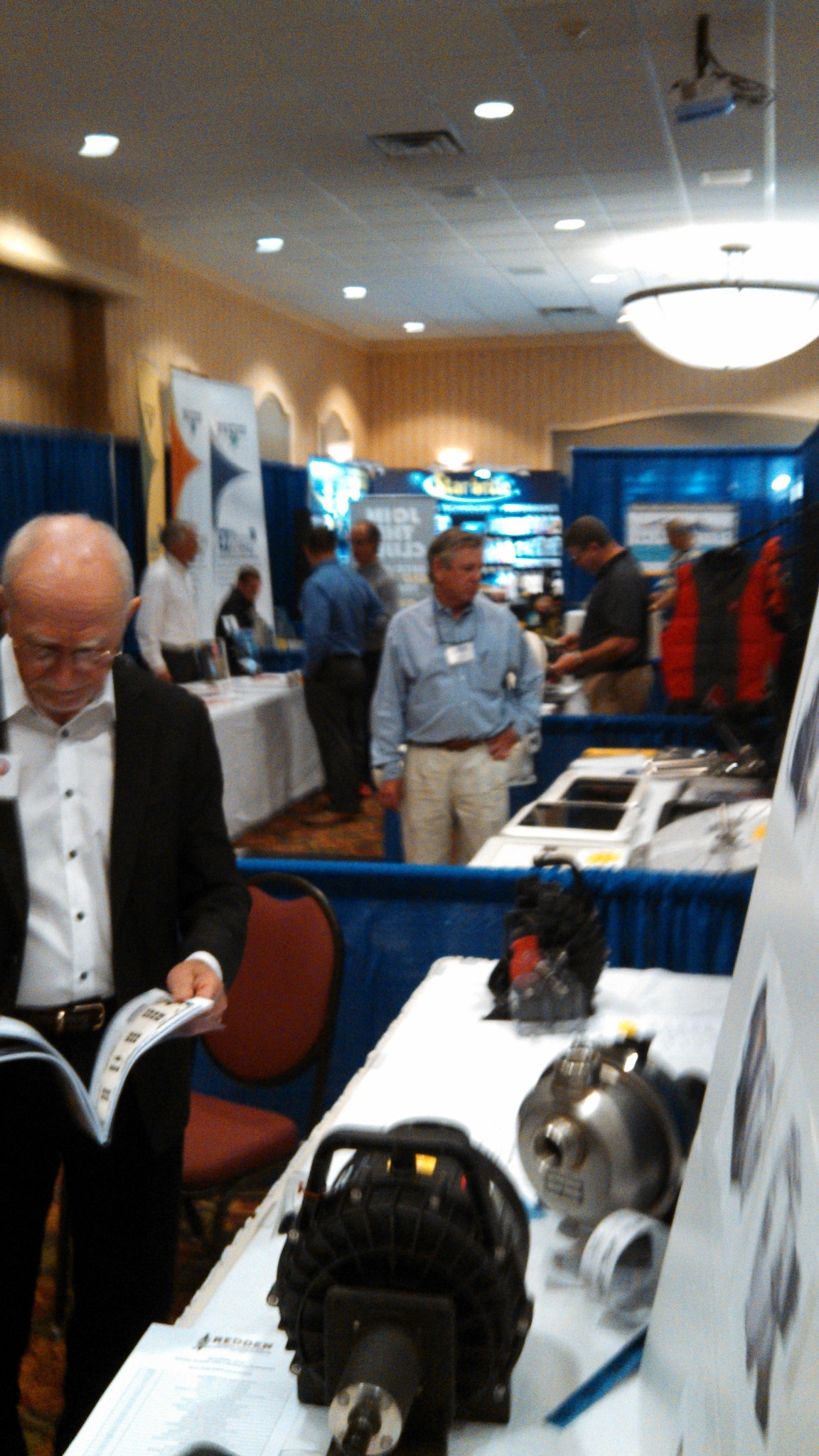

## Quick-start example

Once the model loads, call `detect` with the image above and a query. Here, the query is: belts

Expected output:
[15,997,121,1037]
[439,739,477,752]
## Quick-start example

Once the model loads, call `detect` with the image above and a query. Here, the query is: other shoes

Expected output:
[302,810,354,828]
[301,793,330,813]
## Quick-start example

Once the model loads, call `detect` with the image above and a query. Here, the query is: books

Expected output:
[0,987,214,1145]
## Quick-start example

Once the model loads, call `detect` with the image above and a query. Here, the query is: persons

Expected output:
[0,517,256,1456]
[134,513,701,871]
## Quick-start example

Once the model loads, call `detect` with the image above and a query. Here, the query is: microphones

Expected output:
[272,1120,530,1456]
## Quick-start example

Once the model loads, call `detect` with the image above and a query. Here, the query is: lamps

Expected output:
[615,239,819,373]
[322,413,356,462]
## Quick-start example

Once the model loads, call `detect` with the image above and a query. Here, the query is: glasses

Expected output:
[570,550,585,564]
[350,538,371,546]
[13,641,124,672]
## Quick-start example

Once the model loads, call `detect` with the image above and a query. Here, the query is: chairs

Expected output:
[177,870,344,1265]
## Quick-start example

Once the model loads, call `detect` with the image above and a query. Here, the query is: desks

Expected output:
[466,746,714,870]
[73,952,728,1456]
[185,668,321,841]
